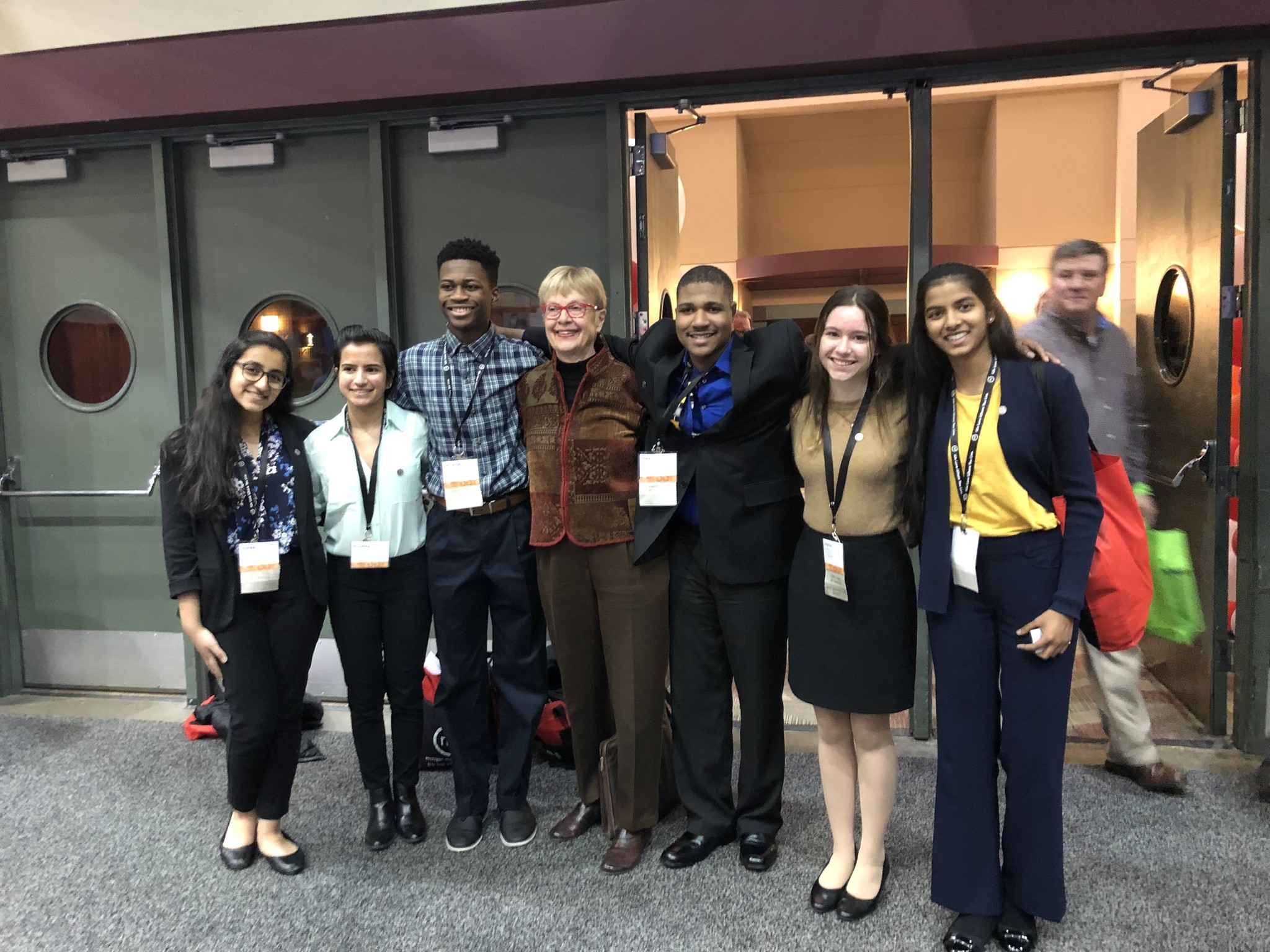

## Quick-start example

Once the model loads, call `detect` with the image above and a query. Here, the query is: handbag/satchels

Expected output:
[415,644,577,771]
[1132,481,1207,645]
[184,689,323,739]
[598,687,678,838]
[1032,357,1154,652]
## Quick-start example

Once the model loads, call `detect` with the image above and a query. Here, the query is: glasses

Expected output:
[234,362,291,390]
[541,302,598,319]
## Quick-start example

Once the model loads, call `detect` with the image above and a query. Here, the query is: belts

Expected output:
[435,494,526,516]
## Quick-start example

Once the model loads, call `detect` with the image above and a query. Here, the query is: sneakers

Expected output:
[446,807,490,851]
[500,807,538,846]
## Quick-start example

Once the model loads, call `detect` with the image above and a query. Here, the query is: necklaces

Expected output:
[832,408,855,428]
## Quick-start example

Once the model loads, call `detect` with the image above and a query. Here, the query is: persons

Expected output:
[160,330,328,875]
[903,262,1105,952]
[495,265,1063,872]
[304,325,432,849]
[1014,239,1187,794]
[515,264,670,875]
[734,284,919,923]
[388,236,549,852]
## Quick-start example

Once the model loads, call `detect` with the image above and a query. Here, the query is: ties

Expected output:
[670,371,717,438]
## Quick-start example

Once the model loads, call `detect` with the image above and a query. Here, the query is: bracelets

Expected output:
[1132,482,1153,495]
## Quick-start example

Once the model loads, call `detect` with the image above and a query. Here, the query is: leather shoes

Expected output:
[997,914,1037,952]
[739,831,778,869]
[1104,759,1185,792]
[395,795,427,843]
[366,799,397,849]
[661,828,737,866]
[811,845,858,913]
[943,914,1000,952]
[600,827,651,873]
[836,853,890,920]
[219,810,258,869]
[548,799,601,841]
[256,830,304,874]
